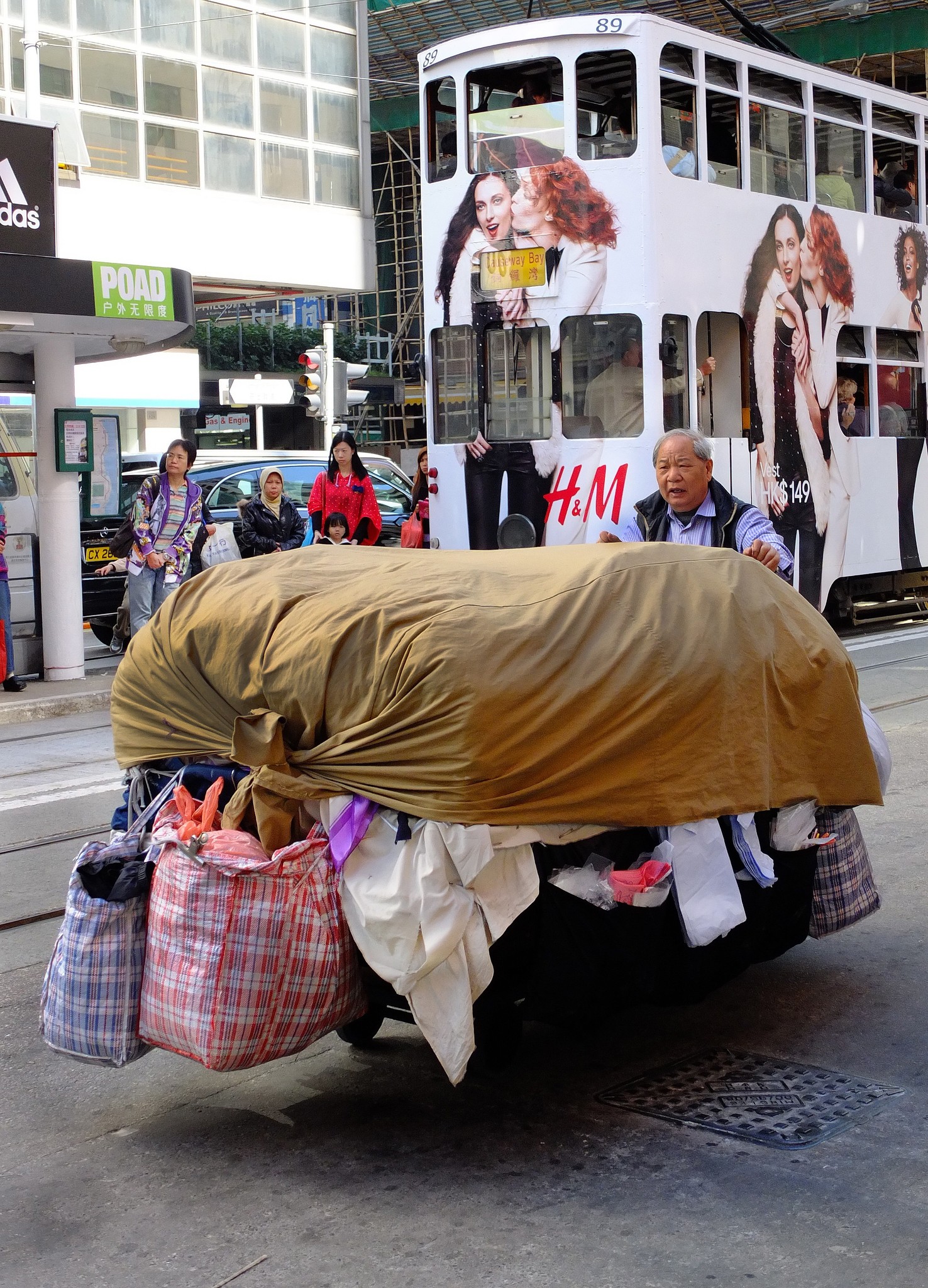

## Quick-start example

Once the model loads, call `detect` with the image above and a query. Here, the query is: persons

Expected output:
[888,222,926,571]
[615,113,921,222]
[0,502,28,693]
[511,79,560,167]
[735,203,854,617]
[307,431,383,547]
[430,129,458,182]
[94,556,130,655]
[410,447,432,549]
[581,337,715,439]
[597,426,798,583]
[500,159,621,547]
[486,138,518,171]
[837,366,915,438]
[235,466,306,558]
[317,511,353,546]
[122,438,205,643]
[158,451,217,578]
[436,167,551,555]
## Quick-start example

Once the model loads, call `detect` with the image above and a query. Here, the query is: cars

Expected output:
[77,448,414,648]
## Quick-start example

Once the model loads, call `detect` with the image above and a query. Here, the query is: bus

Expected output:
[415,12,928,632]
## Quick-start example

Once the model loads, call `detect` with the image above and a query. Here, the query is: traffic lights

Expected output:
[332,357,371,417]
[298,347,326,420]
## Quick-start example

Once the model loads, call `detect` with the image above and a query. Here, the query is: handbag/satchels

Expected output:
[109,473,161,558]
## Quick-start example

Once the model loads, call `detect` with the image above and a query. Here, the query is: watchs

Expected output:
[162,551,170,562]
